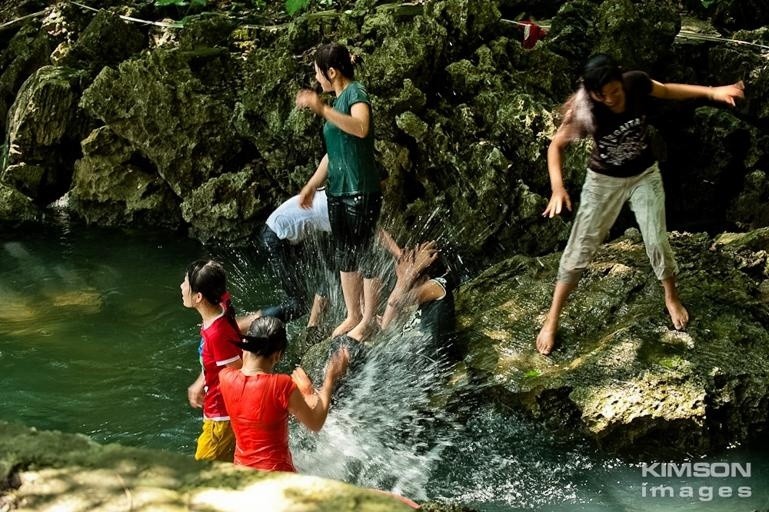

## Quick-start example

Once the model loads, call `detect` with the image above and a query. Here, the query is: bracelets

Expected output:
[408,263,420,281]
[707,83,714,102]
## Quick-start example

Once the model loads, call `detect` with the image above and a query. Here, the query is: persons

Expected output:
[178,259,242,459]
[236,162,408,333]
[293,43,384,339]
[220,315,350,472]
[290,249,455,459]
[316,239,458,398]
[535,53,749,358]
